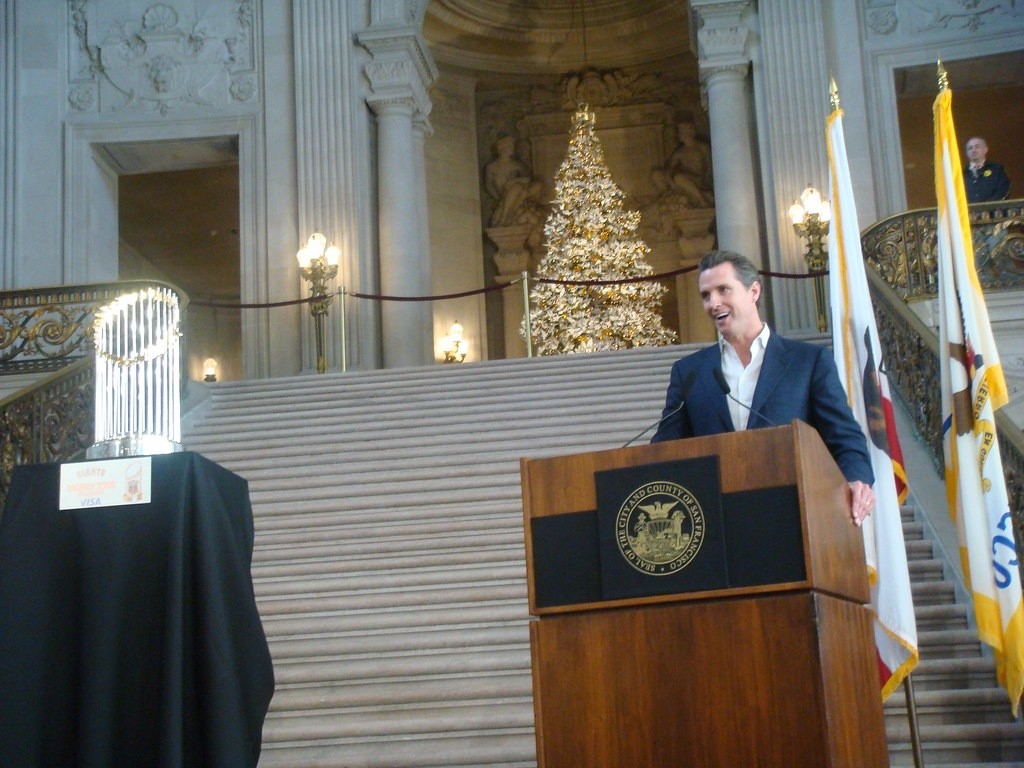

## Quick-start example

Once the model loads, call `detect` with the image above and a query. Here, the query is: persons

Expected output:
[483,134,545,228]
[959,137,1012,291]
[649,249,877,527]
[650,121,712,207]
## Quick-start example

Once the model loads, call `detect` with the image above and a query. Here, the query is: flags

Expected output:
[820,108,920,706]
[930,89,1023,720]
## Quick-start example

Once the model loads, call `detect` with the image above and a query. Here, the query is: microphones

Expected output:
[623,371,698,448]
[713,368,776,426]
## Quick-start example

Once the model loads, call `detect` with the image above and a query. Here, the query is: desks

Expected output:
[1,452,275,768]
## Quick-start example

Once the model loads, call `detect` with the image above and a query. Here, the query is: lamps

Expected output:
[203,358,217,382]
[295,230,341,374]
[438,320,469,363]
[789,185,831,335]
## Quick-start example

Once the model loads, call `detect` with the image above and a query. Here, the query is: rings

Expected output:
[866,501,870,504]
